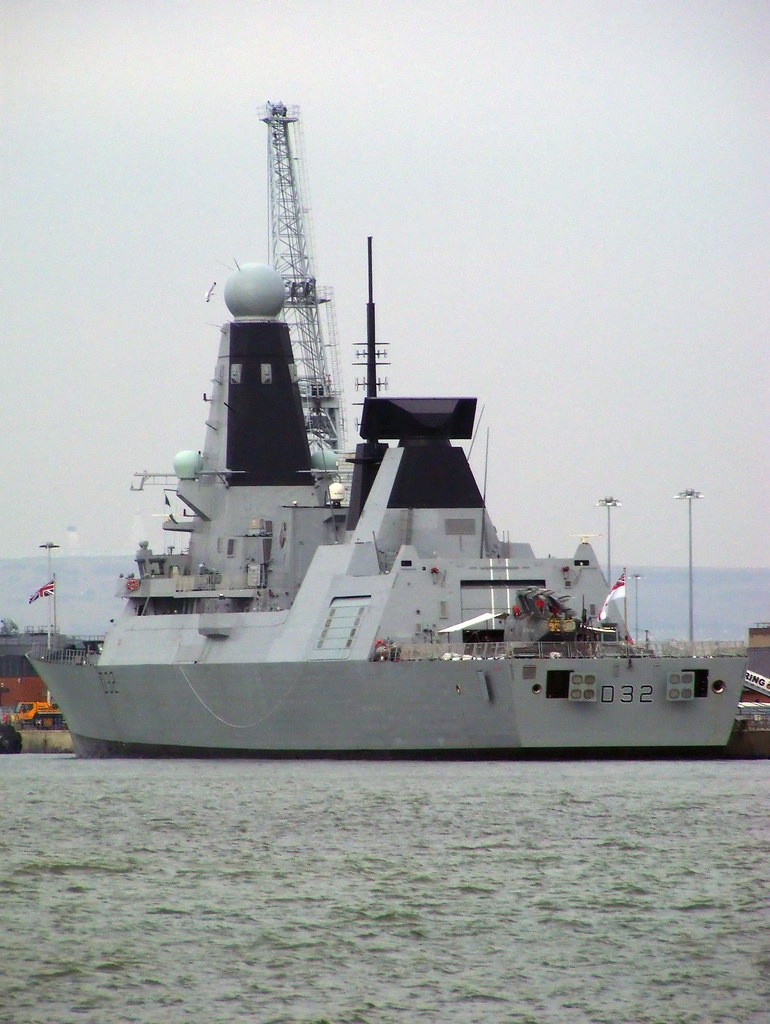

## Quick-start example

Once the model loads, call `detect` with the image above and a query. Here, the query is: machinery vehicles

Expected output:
[6,700,66,730]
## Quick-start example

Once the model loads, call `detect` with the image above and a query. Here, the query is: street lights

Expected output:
[599,497,620,593]
[675,489,703,640]
[38,542,61,651]
[628,574,644,645]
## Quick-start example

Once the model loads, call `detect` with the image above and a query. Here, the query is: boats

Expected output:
[21,100,749,760]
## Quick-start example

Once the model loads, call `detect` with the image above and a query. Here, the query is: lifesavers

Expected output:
[374,640,386,654]
[127,580,138,590]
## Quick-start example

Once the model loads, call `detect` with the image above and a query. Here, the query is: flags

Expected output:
[29,579,54,604]
[597,574,625,621]
